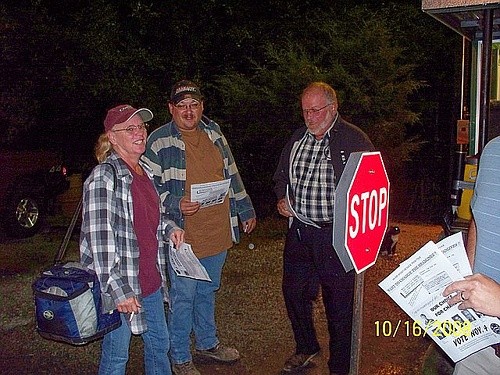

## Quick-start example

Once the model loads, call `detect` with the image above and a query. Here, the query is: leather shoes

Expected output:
[284,349,321,371]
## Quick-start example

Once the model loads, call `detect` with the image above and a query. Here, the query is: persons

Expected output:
[270,80,376,374]
[77,103,188,375]
[442,135,500,375]
[139,77,259,374]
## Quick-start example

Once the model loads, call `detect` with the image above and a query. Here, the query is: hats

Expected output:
[104,104,154,130]
[170,80,202,107]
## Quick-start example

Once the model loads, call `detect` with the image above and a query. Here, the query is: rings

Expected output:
[459,291,466,301]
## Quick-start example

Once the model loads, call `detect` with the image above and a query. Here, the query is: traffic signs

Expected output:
[342,151,391,273]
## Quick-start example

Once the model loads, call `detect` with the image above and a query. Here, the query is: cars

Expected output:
[1,148,68,239]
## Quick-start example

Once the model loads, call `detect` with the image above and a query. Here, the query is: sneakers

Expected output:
[196,343,240,361]
[172,361,201,375]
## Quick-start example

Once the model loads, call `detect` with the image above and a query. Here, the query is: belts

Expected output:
[293,216,334,231]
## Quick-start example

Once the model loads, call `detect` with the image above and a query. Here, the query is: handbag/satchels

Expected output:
[32,261,121,346]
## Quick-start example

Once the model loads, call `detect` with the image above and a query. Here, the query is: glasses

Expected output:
[175,103,202,109]
[111,123,149,133]
[302,103,334,116]
